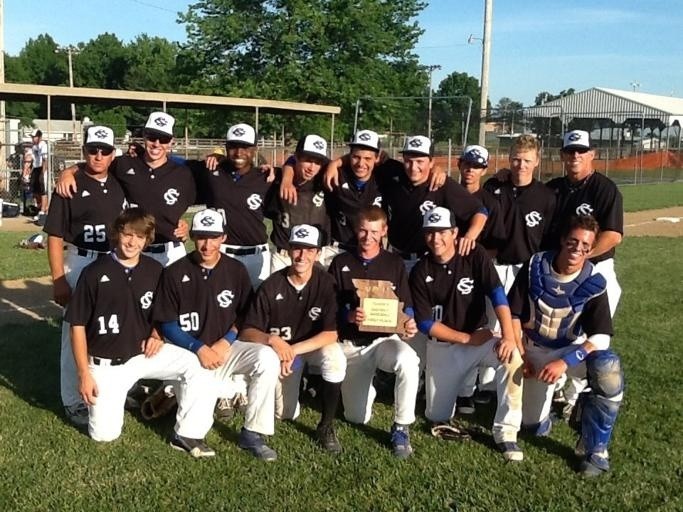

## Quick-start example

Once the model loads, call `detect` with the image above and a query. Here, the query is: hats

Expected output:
[457,145,489,167]
[398,135,432,157]
[84,125,115,149]
[563,129,590,149]
[295,134,330,164]
[145,112,175,136]
[421,206,456,232]
[225,124,257,148]
[189,209,224,236]
[288,223,323,248]
[28,128,42,137]
[349,129,380,153]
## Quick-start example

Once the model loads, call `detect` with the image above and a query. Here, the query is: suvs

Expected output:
[51,137,87,172]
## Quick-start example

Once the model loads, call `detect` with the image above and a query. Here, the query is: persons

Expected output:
[29,129,51,221]
[38,113,623,471]
[17,140,34,201]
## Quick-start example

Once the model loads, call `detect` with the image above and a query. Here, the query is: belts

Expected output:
[226,246,266,255]
[94,356,131,366]
[78,248,106,258]
[277,247,290,256]
[386,241,430,260]
[142,241,180,253]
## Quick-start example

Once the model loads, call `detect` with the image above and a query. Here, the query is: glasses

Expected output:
[561,239,594,256]
[86,146,113,155]
[145,132,171,144]
[562,148,590,155]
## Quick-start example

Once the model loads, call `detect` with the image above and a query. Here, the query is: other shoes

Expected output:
[580,457,603,475]
[128,386,145,407]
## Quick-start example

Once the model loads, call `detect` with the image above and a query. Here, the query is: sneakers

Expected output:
[233,393,248,412]
[237,435,278,462]
[64,400,89,424]
[316,423,342,454]
[390,423,413,457]
[456,395,476,413]
[169,433,216,457]
[475,390,491,403]
[216,396,234,420]
[499,440,524,461]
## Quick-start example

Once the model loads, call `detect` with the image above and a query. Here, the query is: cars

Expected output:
[166,141,197,151]
